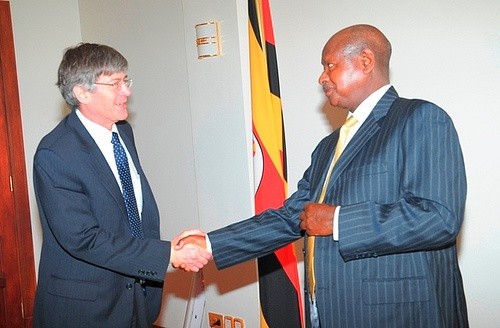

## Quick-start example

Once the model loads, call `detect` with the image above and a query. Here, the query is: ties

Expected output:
[111,131,146,285]
[306,116,358,302]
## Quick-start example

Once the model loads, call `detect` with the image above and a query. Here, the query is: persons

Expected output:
[176,24,469,328]
[30,41,214,328]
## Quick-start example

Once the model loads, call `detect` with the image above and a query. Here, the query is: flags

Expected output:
[247,0,304,328]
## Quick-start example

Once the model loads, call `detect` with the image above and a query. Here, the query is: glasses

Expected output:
[92,79,133,90]
